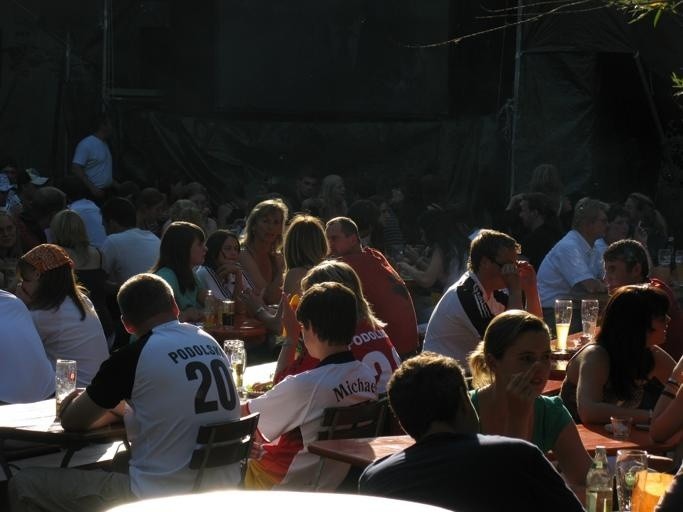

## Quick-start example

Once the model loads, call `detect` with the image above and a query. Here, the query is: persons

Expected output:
[357,351,585,511]
[243,260,404,489]
[560,285,680,425]
[241,283,379,491]
[462,309,595,486]
[8,272,240,509]
[650,357,683,512]
[71,117,114,197]
[0,166,682,403]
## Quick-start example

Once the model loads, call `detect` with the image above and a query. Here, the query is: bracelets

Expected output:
[648,409,655,420]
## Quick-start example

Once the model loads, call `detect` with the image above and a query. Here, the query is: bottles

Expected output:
[586,445,615,512]
[204,290,216,328]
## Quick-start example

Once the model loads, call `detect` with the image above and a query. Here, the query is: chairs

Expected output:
[376,391,396,436]
[309,400,374,497]
[191,413,263,494]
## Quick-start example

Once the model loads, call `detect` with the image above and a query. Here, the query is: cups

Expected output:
[224,339,248,398]
[675,250,683,264]
[657,248,672,266]
[610,416,633,438]
[581,299,600,340]
[55,359,78,422]
[554,299,573,351]
[615,449,648,512]
[222,300,236,329]
